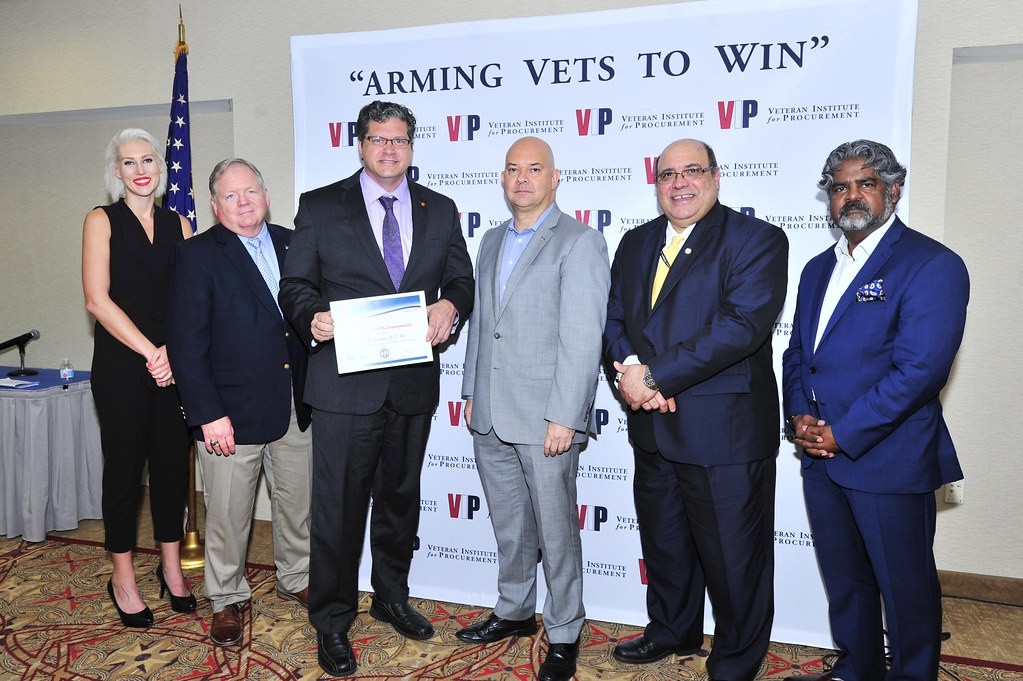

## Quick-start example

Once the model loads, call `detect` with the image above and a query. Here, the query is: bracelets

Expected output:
[789,416,797,433]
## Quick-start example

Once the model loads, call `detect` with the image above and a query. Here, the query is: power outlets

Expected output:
[943,472,966,504]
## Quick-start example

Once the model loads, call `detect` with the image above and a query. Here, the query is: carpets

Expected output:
[0,535,1023,681]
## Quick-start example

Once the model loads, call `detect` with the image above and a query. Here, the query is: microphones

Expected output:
[0,329,40,351]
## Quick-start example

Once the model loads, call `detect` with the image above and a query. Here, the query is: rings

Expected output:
[209,441,218,447]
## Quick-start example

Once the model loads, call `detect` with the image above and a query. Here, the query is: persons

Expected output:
[277,99,476,677]
[782,140,971,681]
[83,127,197,627]
[460,136,611,681]
[602,138,789,681]
[161,157,314,645]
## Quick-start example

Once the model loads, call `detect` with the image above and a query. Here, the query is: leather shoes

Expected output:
[784,670,844,681]
[317,631,357,677]
[537,632,580,681]
[368,595,434,640]
[277,587,309,608]
[613,634,702,664]
[455,612,537,643]
[210,602,242,647]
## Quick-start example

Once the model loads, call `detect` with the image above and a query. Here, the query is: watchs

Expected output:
[643,366,660,391]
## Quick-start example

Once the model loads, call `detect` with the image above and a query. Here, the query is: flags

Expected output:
[160,55,198,233]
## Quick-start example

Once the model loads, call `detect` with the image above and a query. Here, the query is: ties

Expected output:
[247,238,283,321]
[651,234,684,311]
[378,195,405,295]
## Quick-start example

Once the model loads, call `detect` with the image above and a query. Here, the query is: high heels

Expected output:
[157,561,197,613]
[107,577,154,628]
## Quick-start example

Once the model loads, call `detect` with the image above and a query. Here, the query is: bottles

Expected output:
[59,358,75,389]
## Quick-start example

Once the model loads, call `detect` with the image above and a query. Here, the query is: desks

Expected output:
[0,367,103,545]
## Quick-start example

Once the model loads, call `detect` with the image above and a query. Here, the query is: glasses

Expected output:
[656,166,713,183]
[363,135,411,149]
